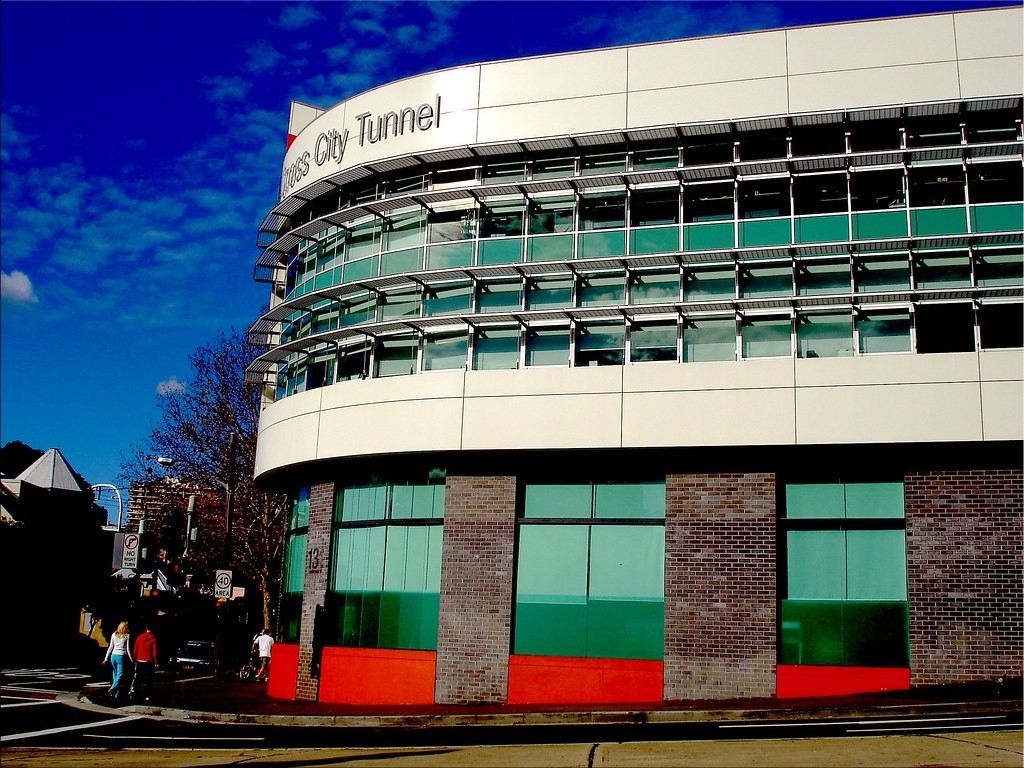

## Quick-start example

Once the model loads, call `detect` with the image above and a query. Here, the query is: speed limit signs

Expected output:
[215,570,233,598]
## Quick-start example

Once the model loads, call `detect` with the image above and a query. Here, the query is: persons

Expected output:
[101,620,160,703]
[251,629,275,682]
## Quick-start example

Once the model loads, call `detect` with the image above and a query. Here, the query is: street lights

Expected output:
[157,457,235,553]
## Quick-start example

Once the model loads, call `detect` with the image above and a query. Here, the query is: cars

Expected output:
[170,641,215,677]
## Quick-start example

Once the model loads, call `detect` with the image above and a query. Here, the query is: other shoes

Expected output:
[265,677,269,682]
[255,674,260,681]
